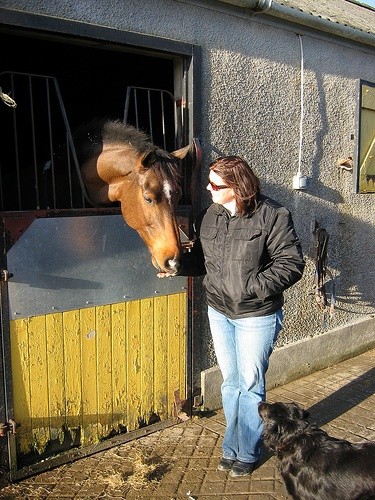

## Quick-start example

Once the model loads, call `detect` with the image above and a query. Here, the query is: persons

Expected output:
[176,155,306,478]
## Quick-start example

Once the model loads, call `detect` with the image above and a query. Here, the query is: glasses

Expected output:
[208,178,231,191]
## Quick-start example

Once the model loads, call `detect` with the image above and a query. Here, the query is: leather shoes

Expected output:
[218,457,235,472]
[229,459,260,477]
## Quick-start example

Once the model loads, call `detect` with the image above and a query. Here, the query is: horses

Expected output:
[82,119,190,278]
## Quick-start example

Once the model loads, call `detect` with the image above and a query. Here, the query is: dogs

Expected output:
[257,400,375,500]
